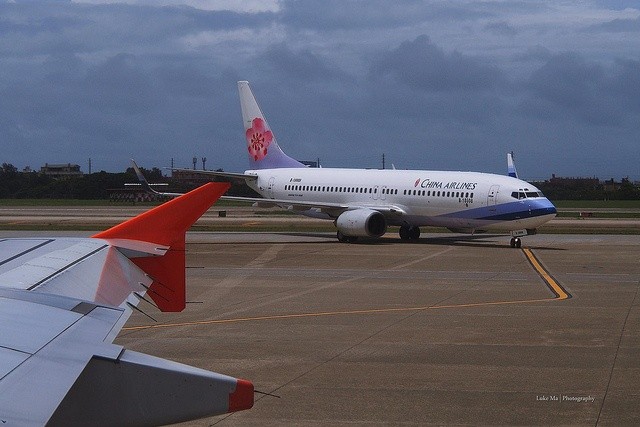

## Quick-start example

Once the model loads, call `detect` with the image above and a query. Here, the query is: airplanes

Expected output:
[0,181,254,427]
[123,80,558,248]
